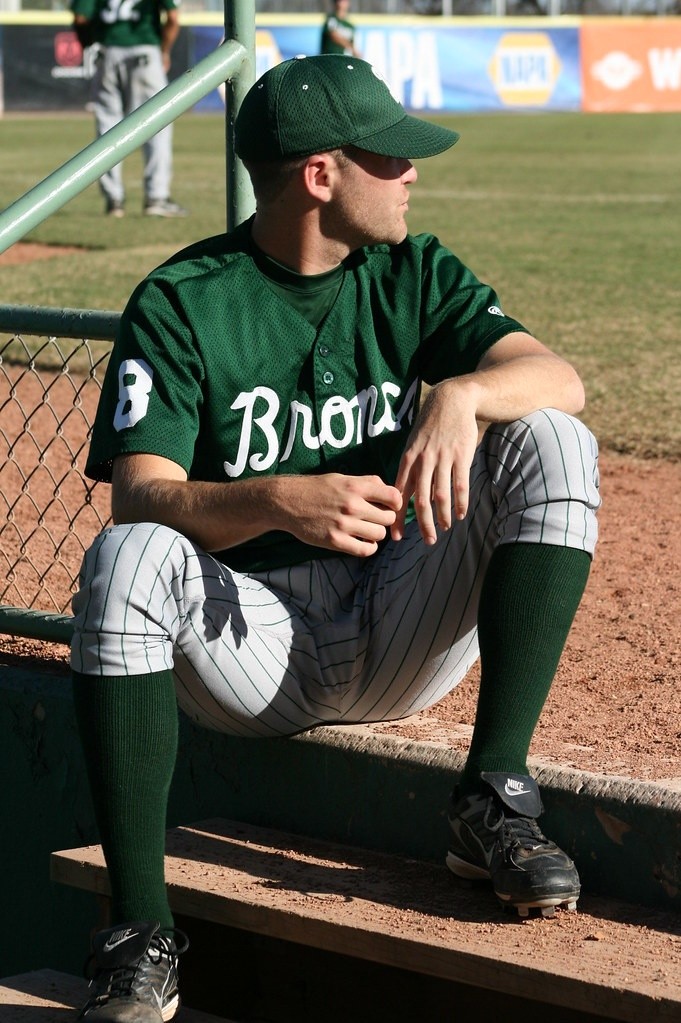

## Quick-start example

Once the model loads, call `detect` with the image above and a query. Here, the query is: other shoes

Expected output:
[106,199,124,218]
[143,197,192,218]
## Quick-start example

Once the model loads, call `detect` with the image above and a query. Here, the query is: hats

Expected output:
[234,54,461,165]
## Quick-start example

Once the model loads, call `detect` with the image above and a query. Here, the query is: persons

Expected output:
[68,0,192,218]
[70,54,600,1023]
[320,0,364,58]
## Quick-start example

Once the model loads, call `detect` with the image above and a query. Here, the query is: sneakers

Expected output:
[74,920,190,1023]
[445,772,581,917]
